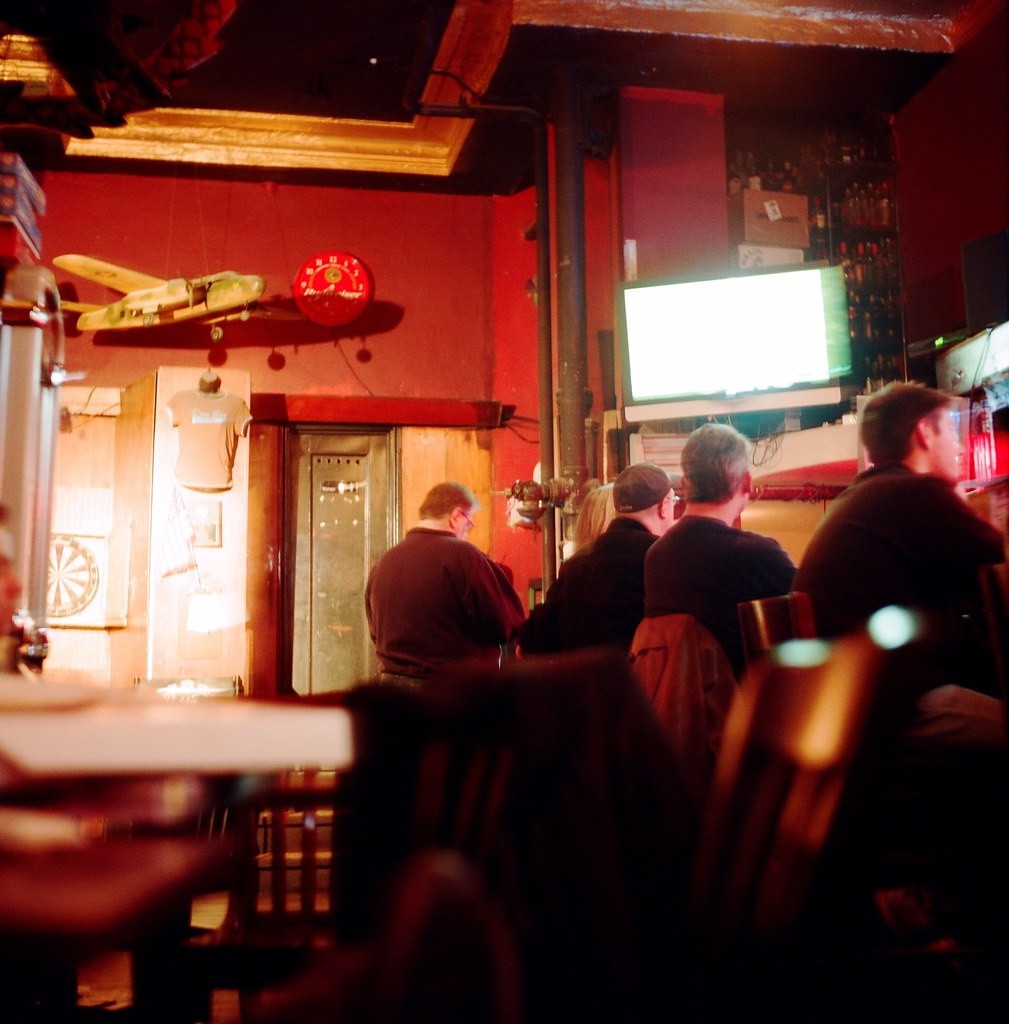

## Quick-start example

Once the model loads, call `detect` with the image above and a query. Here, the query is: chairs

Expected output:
[181,591,1008,1024]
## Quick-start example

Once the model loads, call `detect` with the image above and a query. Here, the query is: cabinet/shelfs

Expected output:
[806,155,910,394]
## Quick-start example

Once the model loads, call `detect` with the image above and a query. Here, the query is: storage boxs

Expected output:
[726,188,813,251]
[729,246,807,270]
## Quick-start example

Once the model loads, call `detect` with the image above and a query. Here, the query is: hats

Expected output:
[613,463,670,513]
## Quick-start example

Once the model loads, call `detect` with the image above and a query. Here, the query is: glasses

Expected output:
[664,496,680,505]
[460,510,475,527]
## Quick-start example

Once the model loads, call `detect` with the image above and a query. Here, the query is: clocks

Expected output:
[290,249,374,330]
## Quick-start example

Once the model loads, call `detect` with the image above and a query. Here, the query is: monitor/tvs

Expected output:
[615,260,840,407]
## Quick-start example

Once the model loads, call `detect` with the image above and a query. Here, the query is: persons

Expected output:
[646,421,797,690]
[361,480,526,689]
[0,548,25,638]
[792,377,1009,750]
[513,461,681,655]
